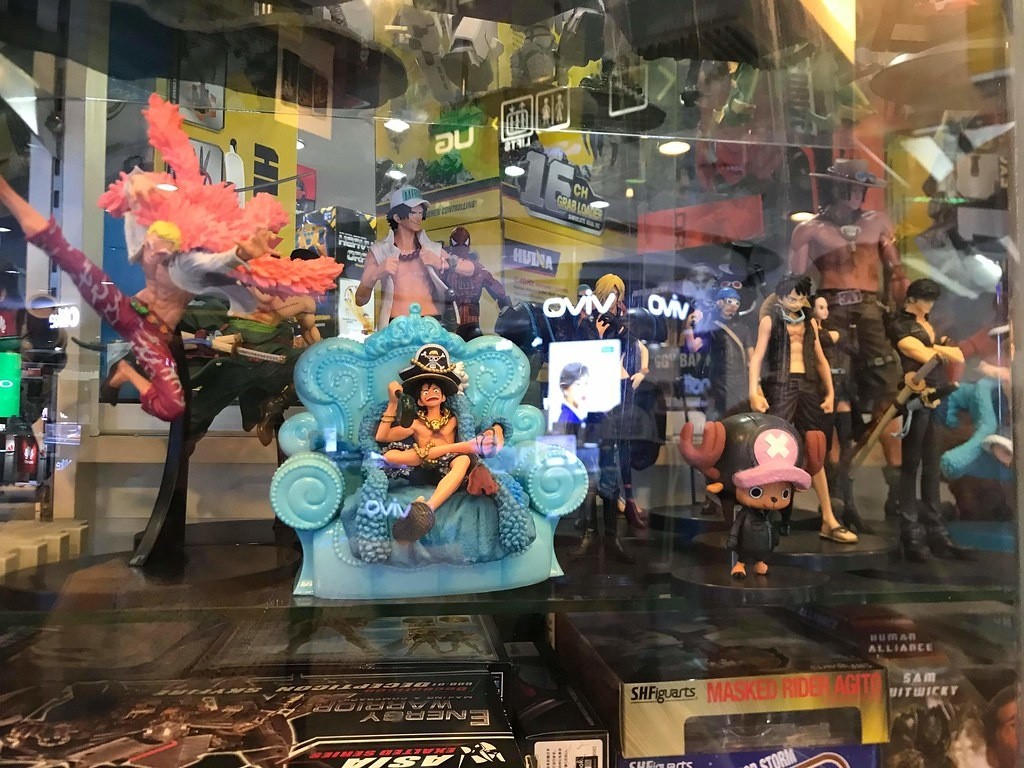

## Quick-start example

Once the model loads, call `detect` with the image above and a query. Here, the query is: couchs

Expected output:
[267,301,590,596]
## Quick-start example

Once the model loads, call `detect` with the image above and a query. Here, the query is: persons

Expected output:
[981,684,1016,768]
[2,158,1013,577]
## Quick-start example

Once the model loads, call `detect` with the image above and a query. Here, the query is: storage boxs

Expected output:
[0,0,1024,768]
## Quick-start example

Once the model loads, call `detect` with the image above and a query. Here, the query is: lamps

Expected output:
[384,95,411,133]
